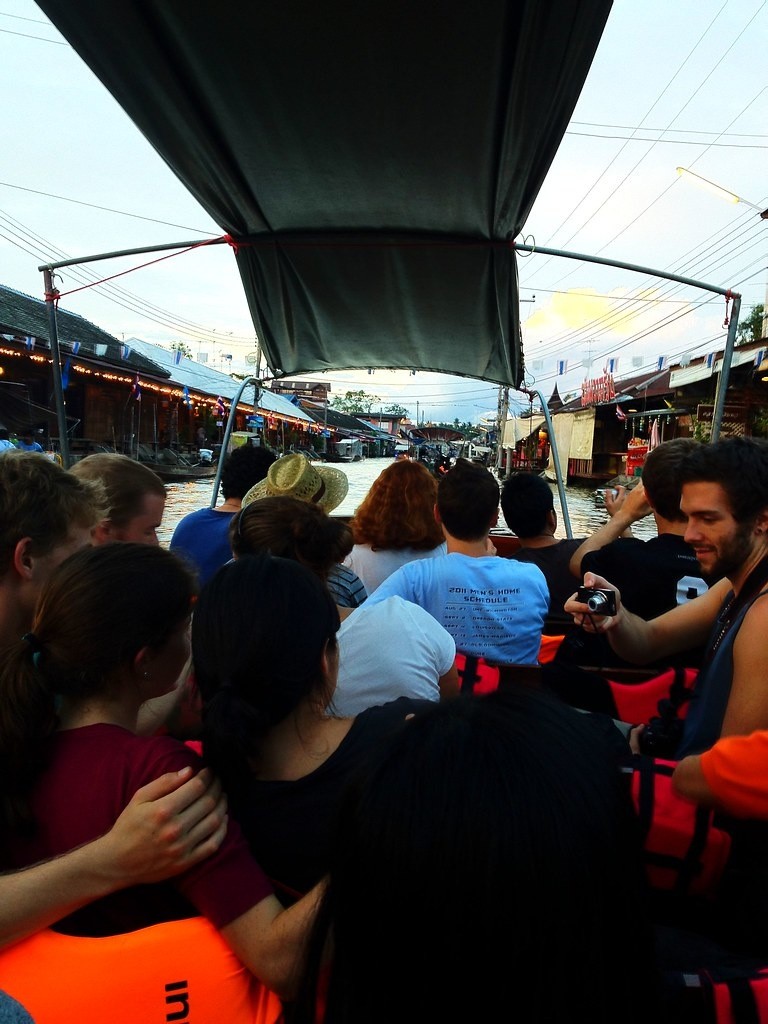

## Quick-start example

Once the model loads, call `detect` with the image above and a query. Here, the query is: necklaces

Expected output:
[710,598,732,652]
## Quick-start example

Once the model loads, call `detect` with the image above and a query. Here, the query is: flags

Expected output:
[0,332,36,351]
[615,405,625,420]
[606,358,619,372]
[704,352,716,368]
[212,396,225,417]
[71,341,81,355]
[556,359,567,375]
[658,355,666,370]
[631,356,643,367]
[679,355,691,367]
[120,345,132,361]
[723,350,741,365]
[94,343,108,356]
[129,372,142,401]
[172,350,185,365]
[754,350,765,367]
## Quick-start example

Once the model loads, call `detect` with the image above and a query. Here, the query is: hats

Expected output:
[241,454,349,514]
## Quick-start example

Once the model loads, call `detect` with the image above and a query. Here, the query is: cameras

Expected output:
[576,586,617,616]
[595,489,618,508]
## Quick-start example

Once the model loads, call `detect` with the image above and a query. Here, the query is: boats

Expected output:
[393,439,492,471]
[334,438,363,463]
[138,461,218,482]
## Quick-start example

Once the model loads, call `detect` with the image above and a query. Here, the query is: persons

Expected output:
[0,424,768,1024]
